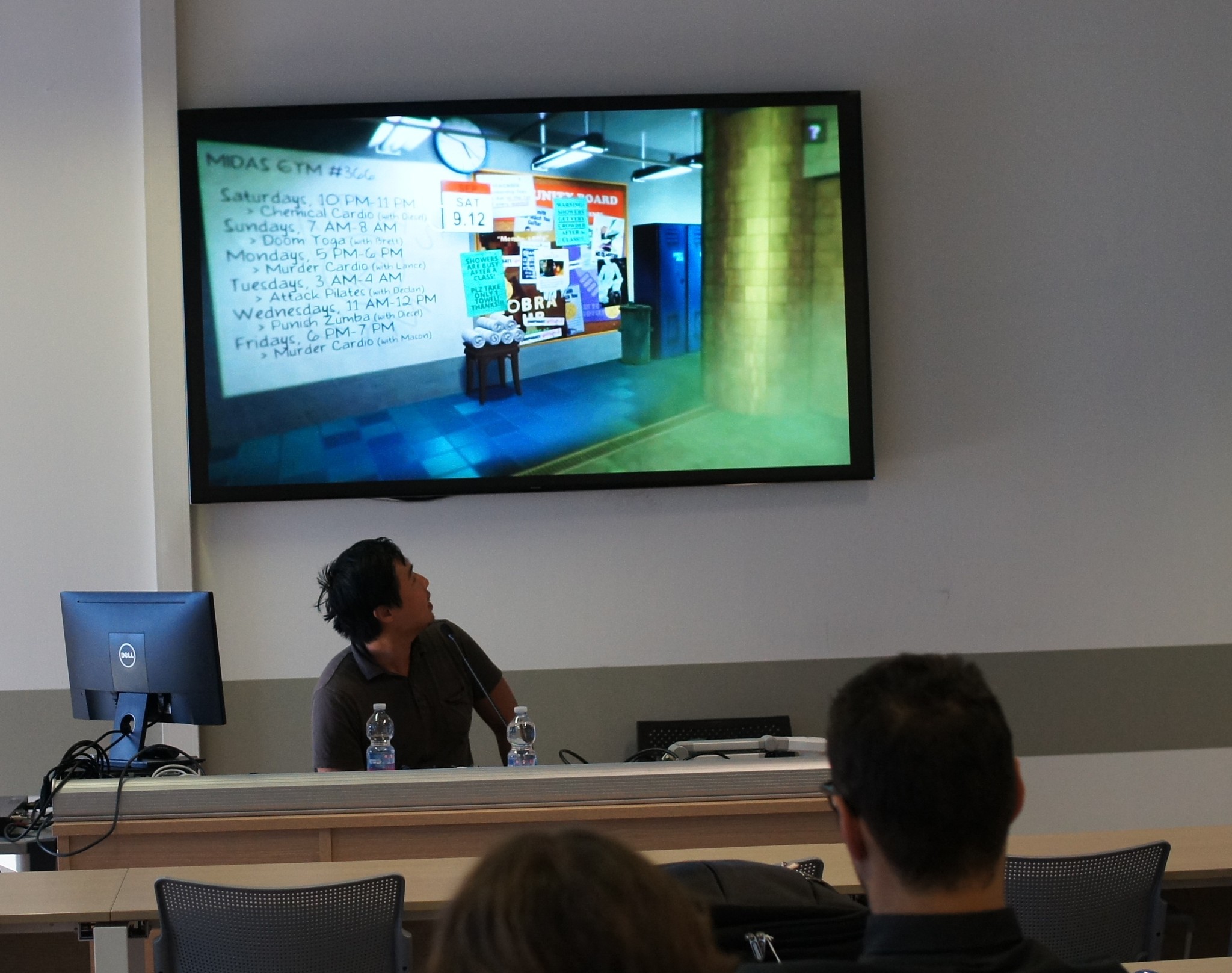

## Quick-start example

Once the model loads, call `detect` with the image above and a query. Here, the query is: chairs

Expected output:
[639,712,800,764]
[1004,840,1171,972]
[154,875,415,973]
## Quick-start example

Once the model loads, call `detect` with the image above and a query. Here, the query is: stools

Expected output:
[464,338,523,405]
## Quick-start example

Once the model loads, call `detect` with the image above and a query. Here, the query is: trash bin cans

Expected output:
[619,301,653,365]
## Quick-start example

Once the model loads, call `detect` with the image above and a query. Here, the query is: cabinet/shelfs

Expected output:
[633,224,702,360]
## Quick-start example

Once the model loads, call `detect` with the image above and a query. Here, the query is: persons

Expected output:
[733,654,1126,973]
[424,829,738,973]
[309,536,542,773]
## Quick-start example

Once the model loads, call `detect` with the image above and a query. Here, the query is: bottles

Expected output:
[366,703,395,770]
[507,706,538,767]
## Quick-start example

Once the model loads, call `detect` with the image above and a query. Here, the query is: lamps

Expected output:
[368,115,441,155]
[631,111,704,183]
[530,111,609,172]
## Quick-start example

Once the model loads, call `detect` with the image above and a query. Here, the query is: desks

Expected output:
[0,799,1232,973]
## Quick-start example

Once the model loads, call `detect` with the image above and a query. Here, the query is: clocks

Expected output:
[432,116,488,175]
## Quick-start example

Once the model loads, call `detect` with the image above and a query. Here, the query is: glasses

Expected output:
[820,781,861,815]
